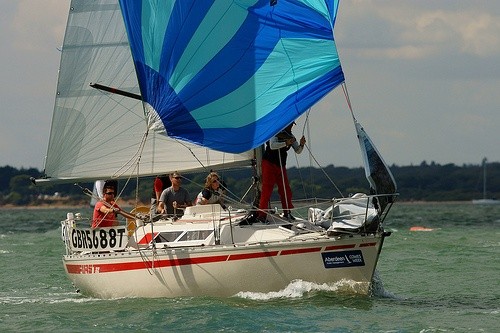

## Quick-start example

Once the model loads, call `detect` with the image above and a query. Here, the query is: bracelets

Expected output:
[113,208,115,212]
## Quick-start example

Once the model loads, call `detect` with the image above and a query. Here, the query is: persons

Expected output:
[158,171,193,220]
[196,172,226,207]
[258,122,306,221]
[92,185,120,227]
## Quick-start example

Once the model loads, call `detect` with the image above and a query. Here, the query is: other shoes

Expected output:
[283,213,295,220]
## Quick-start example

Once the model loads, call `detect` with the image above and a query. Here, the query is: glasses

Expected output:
[213,178,219,183]
[172,177,183,179]
[105,192,115,195]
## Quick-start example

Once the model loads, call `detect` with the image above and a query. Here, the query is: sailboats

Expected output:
[32,1,401,300]
